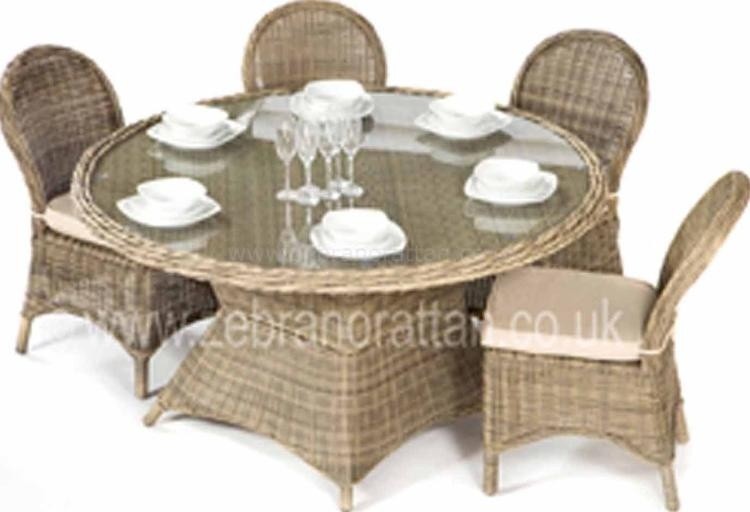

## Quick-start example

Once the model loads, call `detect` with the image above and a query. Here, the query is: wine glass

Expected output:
[273,115,363,202]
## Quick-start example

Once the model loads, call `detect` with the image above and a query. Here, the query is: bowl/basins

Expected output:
[475,158,540,193]
[324,206,389,244]
[138,177,208,213]
[428,97,495,123]
[306,80,365,112]
[162,105,229,137]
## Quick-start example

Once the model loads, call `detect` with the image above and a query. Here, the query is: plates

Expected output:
[115,195,221,230]
[463,170,558,208]
[309,223,407,260]
[415,110,512,142]
[145,120,246,151]
[290,91,375,124]
